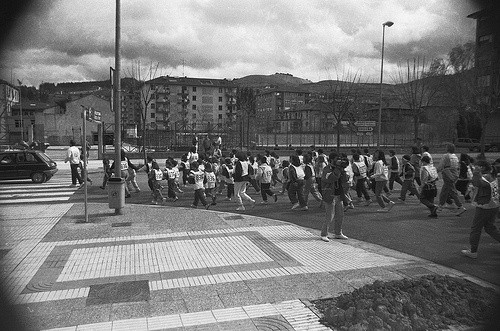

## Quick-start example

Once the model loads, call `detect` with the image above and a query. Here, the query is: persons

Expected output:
[64,140,84,189]
[0,157,7,164]
[78,135,500,260]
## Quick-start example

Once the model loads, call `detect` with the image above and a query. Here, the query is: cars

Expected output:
[0,150,58,183]
[455,138,481,152]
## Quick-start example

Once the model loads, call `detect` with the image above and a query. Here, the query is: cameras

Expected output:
[336,156,350,168]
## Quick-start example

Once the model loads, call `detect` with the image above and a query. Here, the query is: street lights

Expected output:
[377,21,393,148]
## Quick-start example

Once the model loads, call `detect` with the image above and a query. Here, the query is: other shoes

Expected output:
[190,191,309,212]
[90,179,92,186]
[79,182,84,189]
[321,236,329,242]
[319,200,323,208]
[455,207,466,216]
[70,183,77,187]
[461,250,478,259]
[448,203,458,209]
[335,233,348,239]
[152,189,184,205]
[99,186,105,189]
[428,204,439,217]
[344,192,414,213]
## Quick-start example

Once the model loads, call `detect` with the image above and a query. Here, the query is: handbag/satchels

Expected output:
[423,183,437,197]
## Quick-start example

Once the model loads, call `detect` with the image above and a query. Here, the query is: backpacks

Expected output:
[462,160,476,180]
[442,168,459,185]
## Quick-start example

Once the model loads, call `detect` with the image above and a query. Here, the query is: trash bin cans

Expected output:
[107,177,125,209]
